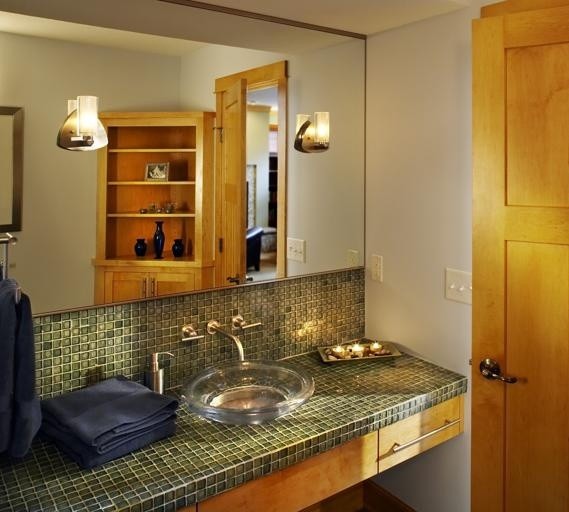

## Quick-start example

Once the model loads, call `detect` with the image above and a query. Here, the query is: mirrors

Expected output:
[0,0,368,319]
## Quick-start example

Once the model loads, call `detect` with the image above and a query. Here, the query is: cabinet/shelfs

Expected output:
[95,110,212,267]
[178,393,466,512]
[93,265,204,304]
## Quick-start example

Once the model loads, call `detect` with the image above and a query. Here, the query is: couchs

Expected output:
[245,225,263,271]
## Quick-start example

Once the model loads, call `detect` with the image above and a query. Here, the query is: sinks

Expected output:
[181,359,315,424]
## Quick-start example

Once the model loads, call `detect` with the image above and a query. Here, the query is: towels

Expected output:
[41,376,179,452]
[40,420,177,472]
[0,278,42,466]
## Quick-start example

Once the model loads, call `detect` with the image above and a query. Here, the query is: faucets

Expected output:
[207,320,244,362]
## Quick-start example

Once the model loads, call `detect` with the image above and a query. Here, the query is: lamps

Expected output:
[66,100,77,116]
[57,95,110,154]
[292,112,331,153]
[295,112,310,135]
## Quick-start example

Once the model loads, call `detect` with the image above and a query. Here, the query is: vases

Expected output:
[154,221,165,260]
[171,238,184,257]
[134,237,147,257]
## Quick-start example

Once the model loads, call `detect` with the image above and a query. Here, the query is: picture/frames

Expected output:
[1,106,24,234]
[143,162,169,182]
